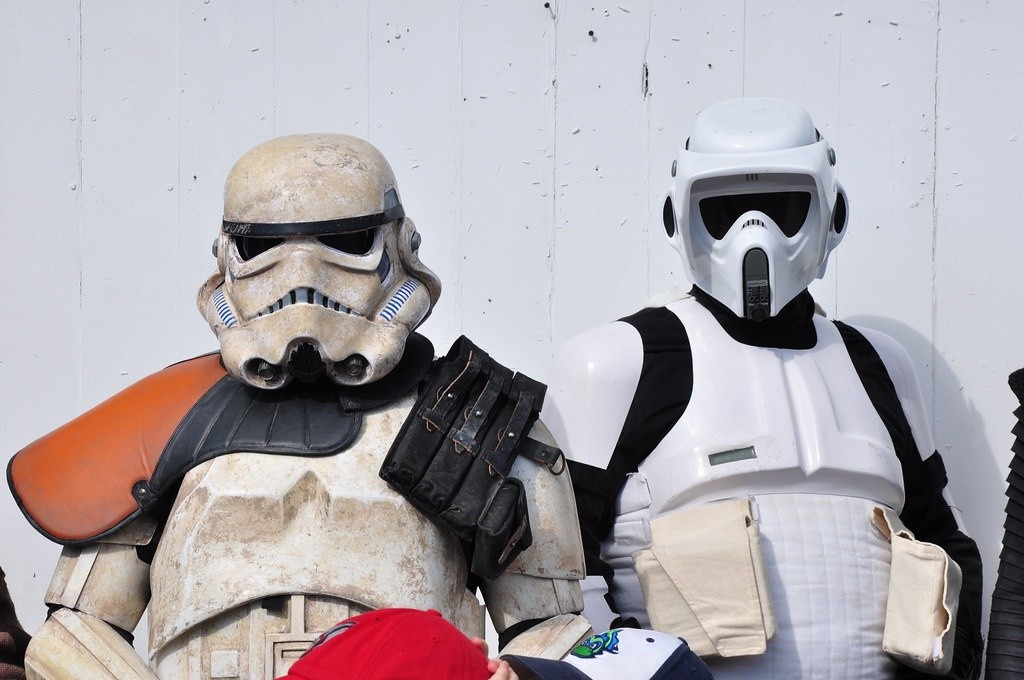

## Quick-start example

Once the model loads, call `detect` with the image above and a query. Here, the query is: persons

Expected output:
[274,608,521,680]
[7,133,585,680]
[534,97,982,680]
[501,628,716,680]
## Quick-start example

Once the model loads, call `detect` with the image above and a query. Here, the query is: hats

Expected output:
[500,627,712,680]
[275,607,493,680]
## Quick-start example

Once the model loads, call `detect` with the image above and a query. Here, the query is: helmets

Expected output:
[195,132,440,391]
[663,100,849,322]
